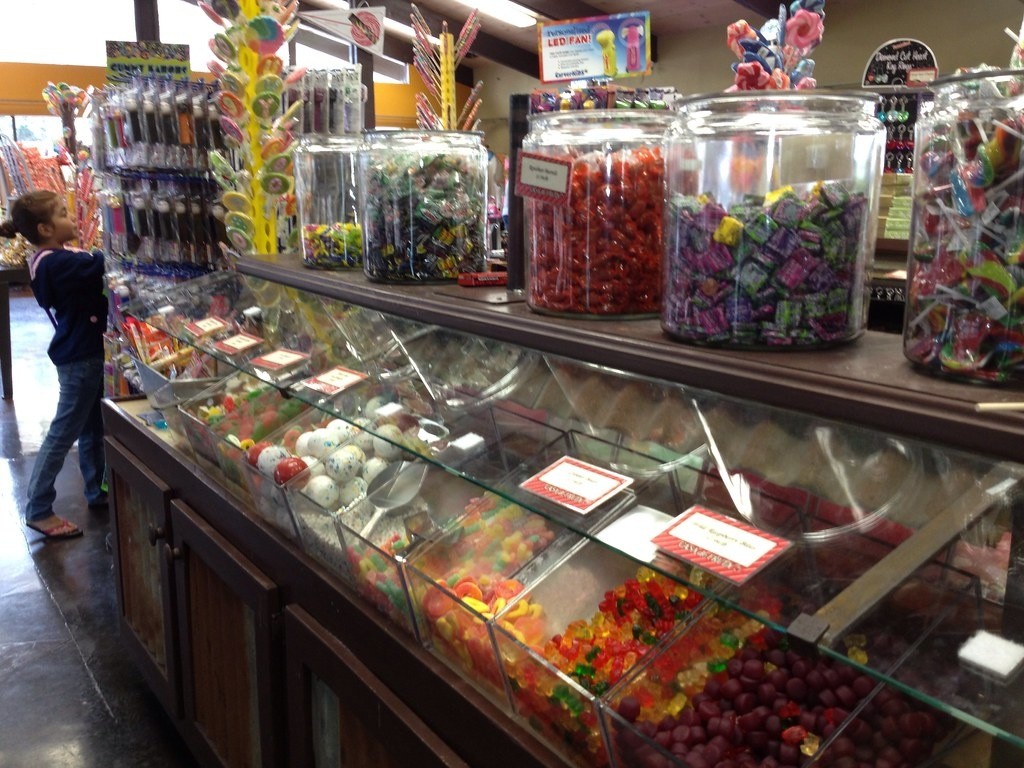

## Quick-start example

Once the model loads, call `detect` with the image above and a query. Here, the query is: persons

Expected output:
[0,191,109,539]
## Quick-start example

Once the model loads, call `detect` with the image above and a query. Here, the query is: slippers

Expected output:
[26,519,83,538]
[88,496,109,509]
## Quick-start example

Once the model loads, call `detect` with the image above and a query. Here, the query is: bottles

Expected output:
[360,130,489,285]
[902,68,1024,392]
[522,109,677,320]
[662,90,888,353]
[293,134,364,272]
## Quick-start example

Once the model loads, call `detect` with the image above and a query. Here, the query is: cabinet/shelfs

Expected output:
[97,397,578,768]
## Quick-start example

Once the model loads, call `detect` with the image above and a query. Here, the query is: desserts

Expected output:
[181,385,945,768]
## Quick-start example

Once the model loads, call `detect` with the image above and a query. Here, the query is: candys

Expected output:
[199,0,1024,381]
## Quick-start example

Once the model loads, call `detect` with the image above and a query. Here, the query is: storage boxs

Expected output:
[177,361,1024,768]
